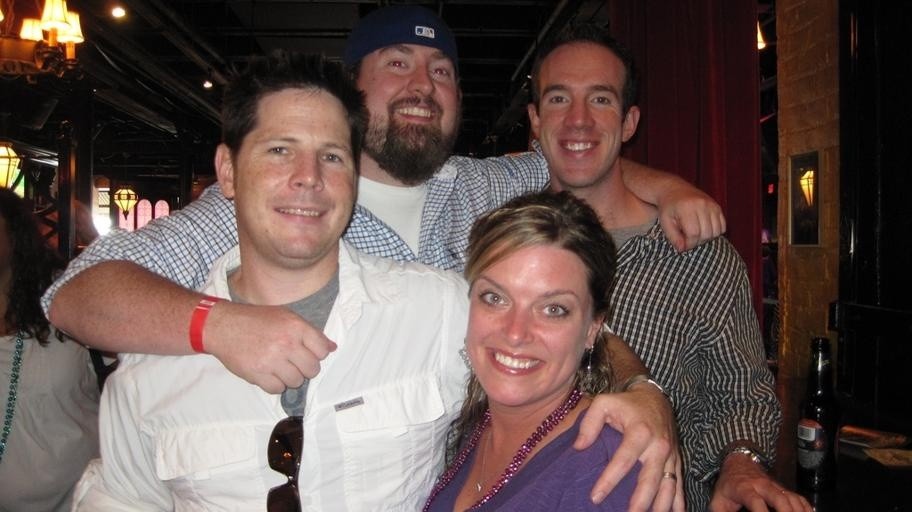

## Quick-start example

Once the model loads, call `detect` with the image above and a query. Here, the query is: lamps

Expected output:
[19,0,86,79]
[114,182,138,221]
[0,142,23,190]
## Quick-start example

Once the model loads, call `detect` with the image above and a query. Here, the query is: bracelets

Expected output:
[188,291,219,356]
[719,439,777,474]
[619,370,670,395]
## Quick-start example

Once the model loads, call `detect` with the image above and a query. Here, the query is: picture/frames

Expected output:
[786,148,823,249]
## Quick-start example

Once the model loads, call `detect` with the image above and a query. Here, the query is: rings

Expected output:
[780,488,788,496]
[662,472,678,483]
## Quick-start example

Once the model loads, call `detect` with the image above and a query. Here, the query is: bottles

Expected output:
[794,334,837,496]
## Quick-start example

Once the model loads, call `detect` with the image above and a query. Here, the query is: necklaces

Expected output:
[0,333,24,450]
[420,382,583,512]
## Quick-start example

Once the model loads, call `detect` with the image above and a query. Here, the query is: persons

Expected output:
[1,186,104,511]
[36,165,99,260]
[69,42,688,512]
[525,20,817,512]
[37,0,730,397]
[417,188,671,512]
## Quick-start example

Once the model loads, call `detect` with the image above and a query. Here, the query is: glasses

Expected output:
[266,413,303,511]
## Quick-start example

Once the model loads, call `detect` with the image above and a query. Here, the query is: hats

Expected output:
[342,9,459,66]
